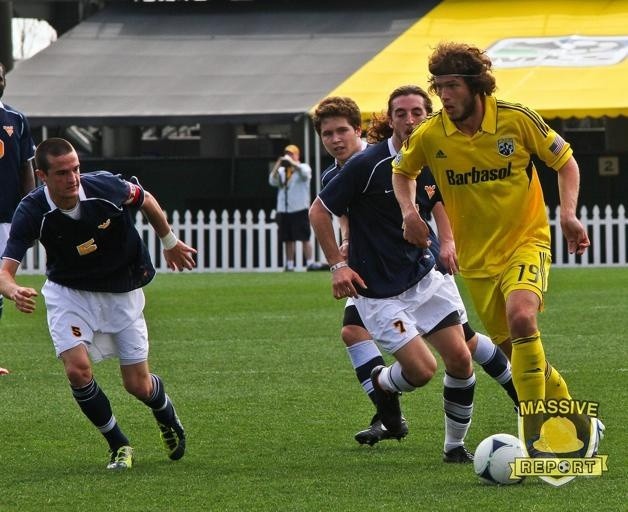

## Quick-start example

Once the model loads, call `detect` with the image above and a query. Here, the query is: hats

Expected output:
[286,145,298,152]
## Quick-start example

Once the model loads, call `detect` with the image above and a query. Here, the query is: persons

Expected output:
[268,144,319,272]
[307,84,477,465]
[0,136,199,469]
[0,61,37,377]
[311,95,521,447]
[390,41,607,459]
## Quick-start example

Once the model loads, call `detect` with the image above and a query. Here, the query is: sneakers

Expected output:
[442,447,475,468]
[578,416,605,475]
[370,365,402,431]
[106,446,134,471]
[525,439,558,471]
[354,412,410,448]
[152,392,185,461]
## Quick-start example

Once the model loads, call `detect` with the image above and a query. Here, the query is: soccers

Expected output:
[473,432,528,486]
[558,460,571,473]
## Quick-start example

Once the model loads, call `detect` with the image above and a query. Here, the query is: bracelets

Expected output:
[342,238,349,243]
[329,260,348,273]
